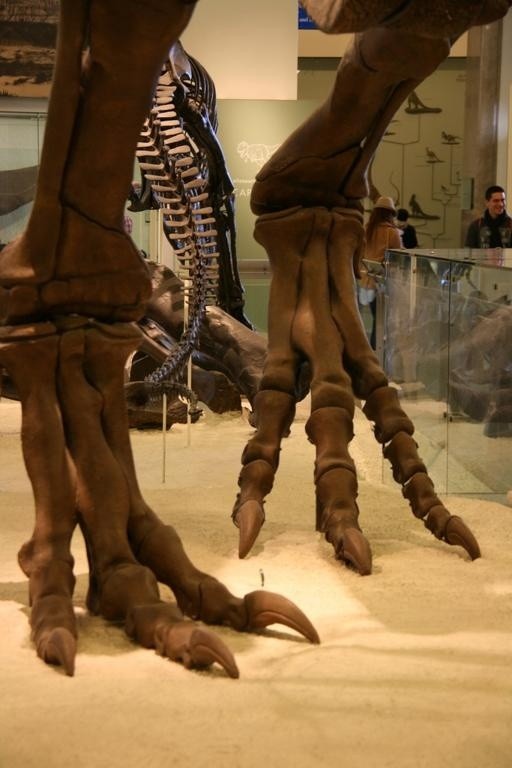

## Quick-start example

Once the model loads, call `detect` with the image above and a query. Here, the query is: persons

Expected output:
[358,195,401,350]
[394,207,419,251]
[463,185,511,249]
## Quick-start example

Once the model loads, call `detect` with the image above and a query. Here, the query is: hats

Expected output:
[373,194,398,219]
